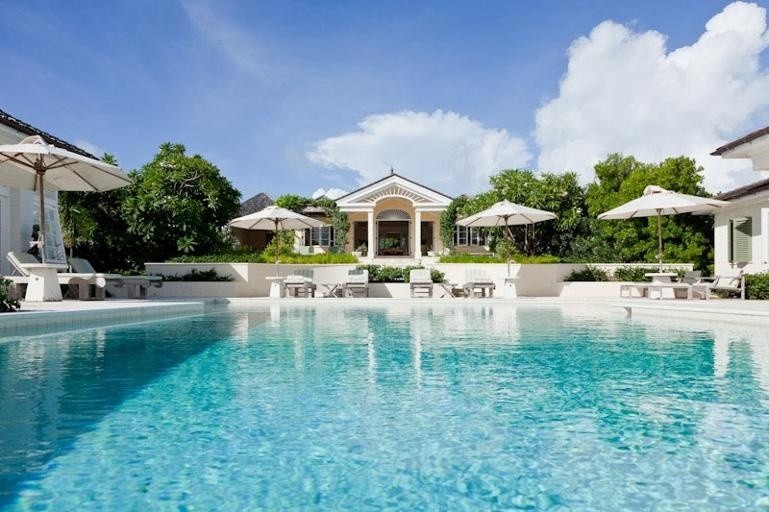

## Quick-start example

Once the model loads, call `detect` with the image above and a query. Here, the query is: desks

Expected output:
[644,272,679,299]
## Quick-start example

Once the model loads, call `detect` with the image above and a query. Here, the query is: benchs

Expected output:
[618,280,690,299]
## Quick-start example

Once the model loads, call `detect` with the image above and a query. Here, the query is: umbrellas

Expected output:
[597,183,729,273]
[455,199,556,276]
[228,204,326,277]
[0,139,135,263]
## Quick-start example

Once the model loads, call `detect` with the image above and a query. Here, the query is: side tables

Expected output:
[436,284,458,299]
[322,282,341,298]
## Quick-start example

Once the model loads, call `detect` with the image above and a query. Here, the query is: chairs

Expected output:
[409,268,435,297]
[283,268,318,299]
[344,269,369,298]
[0,251,165,302]
[686,269,747,301]
[462,268,496,297]
